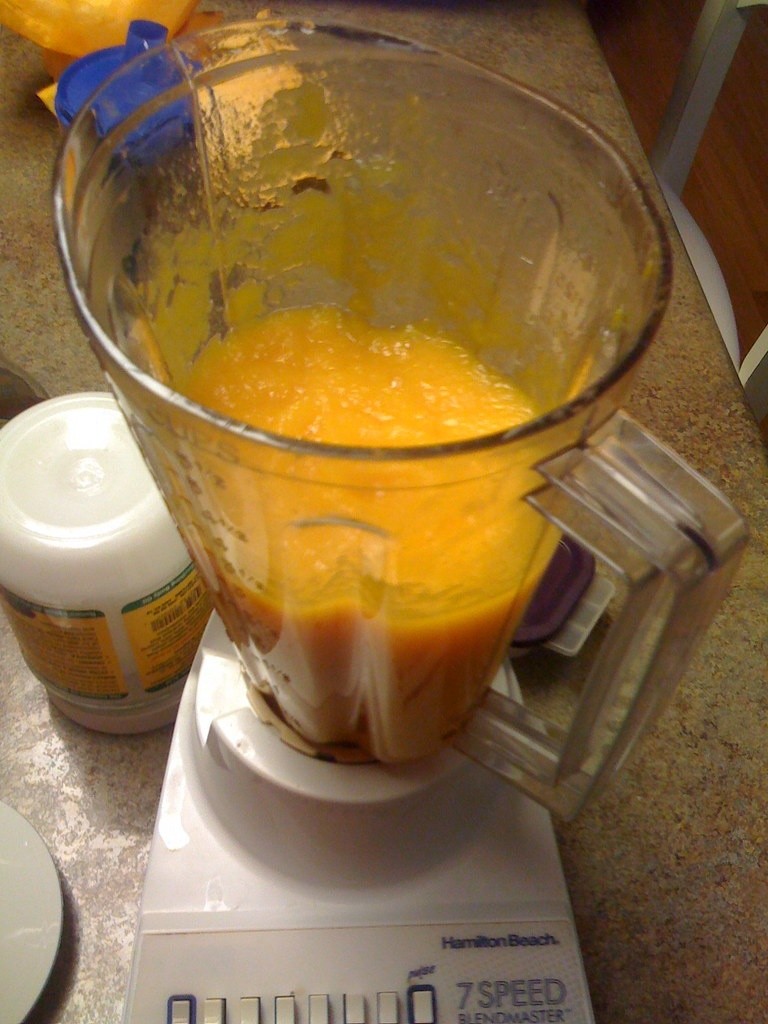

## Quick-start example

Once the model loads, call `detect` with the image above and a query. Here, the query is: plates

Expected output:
[0,801,64,1024]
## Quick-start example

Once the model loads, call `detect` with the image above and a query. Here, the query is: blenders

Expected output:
[52,16,751,1024]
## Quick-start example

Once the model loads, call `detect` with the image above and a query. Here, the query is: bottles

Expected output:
[0,388,209,736]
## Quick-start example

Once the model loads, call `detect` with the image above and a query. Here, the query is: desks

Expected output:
[0,0,768,1024]
